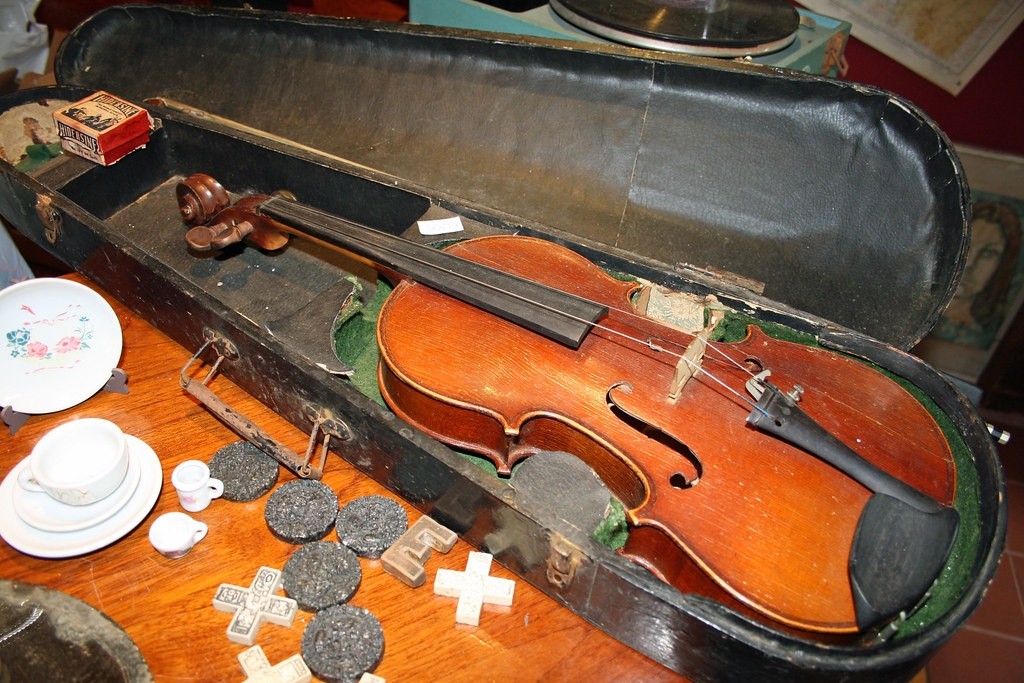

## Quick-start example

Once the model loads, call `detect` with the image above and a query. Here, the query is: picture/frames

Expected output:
[917,144,1024,384]
[798,0,1024,96]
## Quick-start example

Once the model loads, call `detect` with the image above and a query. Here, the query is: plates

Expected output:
[0,433,162,561]
[0,277,123,415]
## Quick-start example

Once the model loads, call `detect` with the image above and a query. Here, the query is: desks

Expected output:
[2,273,928,682]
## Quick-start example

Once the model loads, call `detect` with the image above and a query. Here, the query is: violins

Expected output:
[173,171,960,646]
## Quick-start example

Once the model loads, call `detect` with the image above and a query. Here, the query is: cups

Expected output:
[149,512,208,558]
[172,459,224,512]
[18,417,130,506]
